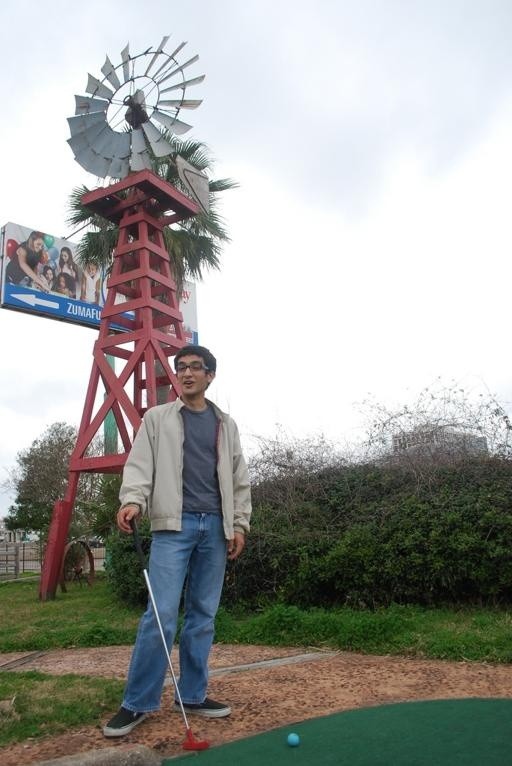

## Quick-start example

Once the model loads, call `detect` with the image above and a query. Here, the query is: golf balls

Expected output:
[287,733,299,746]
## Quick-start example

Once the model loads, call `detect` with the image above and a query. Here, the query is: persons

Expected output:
[101,344,254,738]
[5,230,101,304]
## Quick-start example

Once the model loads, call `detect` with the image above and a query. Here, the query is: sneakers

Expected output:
[173,696,231,718]
[103,705,148,738]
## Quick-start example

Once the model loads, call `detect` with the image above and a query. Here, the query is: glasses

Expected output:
[176,362,210,372]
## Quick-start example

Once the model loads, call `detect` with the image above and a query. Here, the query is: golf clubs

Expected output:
[129,516,208,750]
